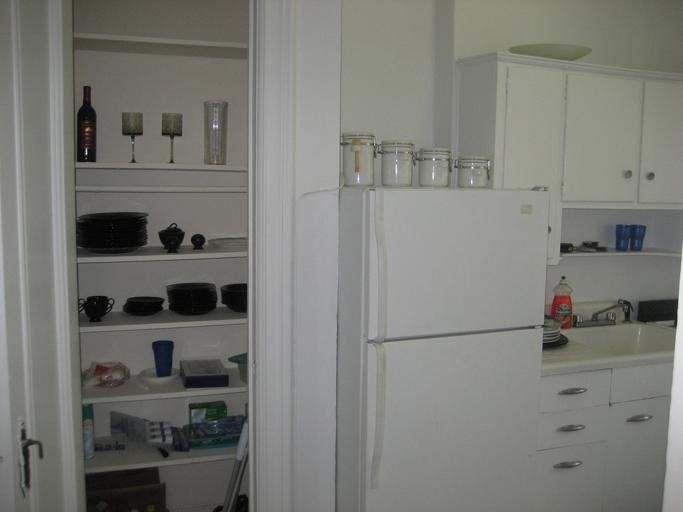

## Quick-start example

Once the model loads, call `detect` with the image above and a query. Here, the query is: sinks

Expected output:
[559,320,676,361]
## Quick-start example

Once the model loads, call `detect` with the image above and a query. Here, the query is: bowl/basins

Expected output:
[158,222,186,250]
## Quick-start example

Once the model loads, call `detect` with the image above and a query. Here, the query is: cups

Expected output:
[615,224,631,251]
[630,225,646,251]
[83,296,115,323]
[151,340,174,376]
[203,100,229,165]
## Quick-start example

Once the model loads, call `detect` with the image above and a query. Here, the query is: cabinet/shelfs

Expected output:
[70,0,250,512]
[562,68,681,212]
[451,60,568,269]
[533,356,673,512]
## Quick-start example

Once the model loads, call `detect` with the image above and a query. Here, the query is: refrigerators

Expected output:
[339,186,551,512]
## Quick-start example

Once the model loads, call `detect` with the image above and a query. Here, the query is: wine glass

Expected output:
[121,111,143,164]
[161,112,183,163]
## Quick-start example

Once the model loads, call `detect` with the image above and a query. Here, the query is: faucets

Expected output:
[591,302,628,319]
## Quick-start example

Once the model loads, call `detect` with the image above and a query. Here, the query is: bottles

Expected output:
[379,139,416,188]
[415,147,453,188]
[82,403,95,464]
[340,132,377,188]
[454,155,492,189]
[76,85,97,163]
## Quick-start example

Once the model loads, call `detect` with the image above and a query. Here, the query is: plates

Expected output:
[76,211,149,254]
[138,367,180,384]
[208,237,248,253]
[509,43,592,61]
[123,297,165,317]
[167,282,218,316]
[220,283,247,314]
[543,317,568,349]
